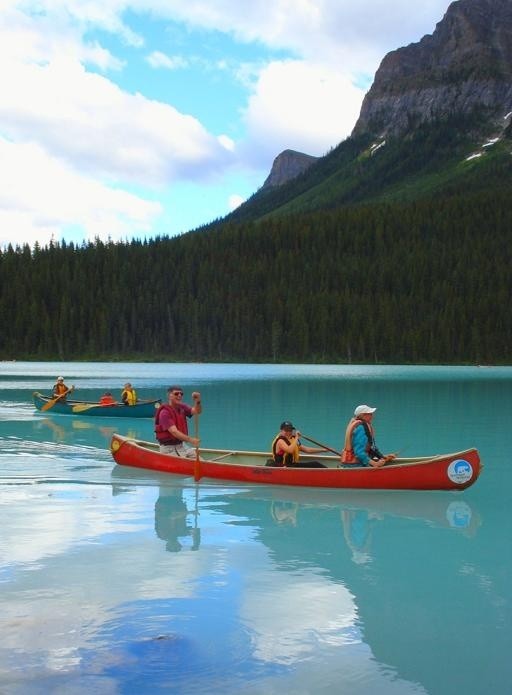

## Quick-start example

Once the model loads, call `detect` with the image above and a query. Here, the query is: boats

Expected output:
[111,433,483,490]
[32,391,162,418]
[223,487,480,540]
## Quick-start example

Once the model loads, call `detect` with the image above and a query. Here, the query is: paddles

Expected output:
[73,403,126,412]
[194,398,200,482]
[42,388,74,411]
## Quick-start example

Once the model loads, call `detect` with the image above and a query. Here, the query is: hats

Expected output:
[354,405,377,417]
[280,421,295,429]
[58,377,63,380]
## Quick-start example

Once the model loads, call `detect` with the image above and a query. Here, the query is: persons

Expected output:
[334,509,386,586]
[270,419,332,468]
[153,387,203,463]
[338,403,396,468]
[101,392,118,408]
[261,500,302,532]
[51,376,73,403]
[121,383,150,405]
[146,480,201,554]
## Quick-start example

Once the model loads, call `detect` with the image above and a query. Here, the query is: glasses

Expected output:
[171,391,184,396]
